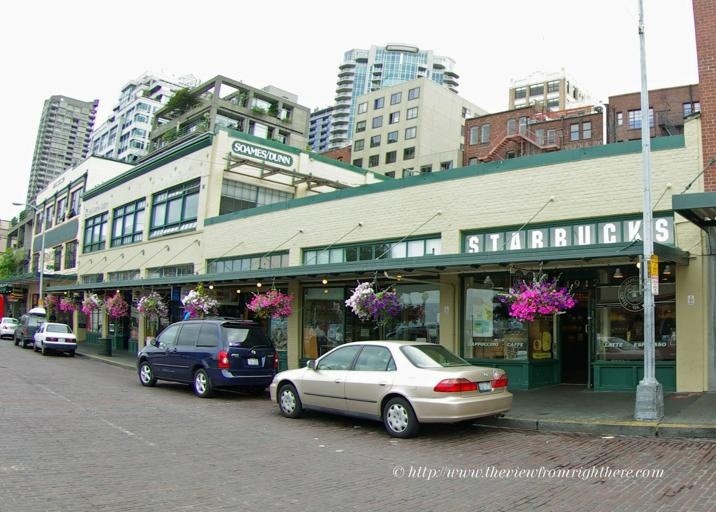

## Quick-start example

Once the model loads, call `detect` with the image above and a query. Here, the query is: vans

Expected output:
[132,315,280,399]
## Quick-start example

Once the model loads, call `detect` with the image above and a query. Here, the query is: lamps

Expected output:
[612,268,623,278]
[663,265,672,274]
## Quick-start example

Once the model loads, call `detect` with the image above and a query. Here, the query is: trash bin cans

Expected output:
[97,338,112,357]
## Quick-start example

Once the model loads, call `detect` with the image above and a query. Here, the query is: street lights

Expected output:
[10,201,47,307]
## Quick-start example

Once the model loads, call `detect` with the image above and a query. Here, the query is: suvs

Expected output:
[12,314,47,350]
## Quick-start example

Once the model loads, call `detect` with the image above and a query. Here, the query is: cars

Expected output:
[30,322,78,356]
[0,315,19,340]
[264,337,515,442]
[98,323,113,334]
[385,323,439,343]
[595,335,675,360]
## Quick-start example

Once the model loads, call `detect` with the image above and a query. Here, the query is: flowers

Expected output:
[344,282,401,322]
[137,292,169,319]
[43,294,58,311]
[104,290,129,318]
[498,271,579,322]
[245,289,295,319]
[180,283,220,318]
[81,294,97,315]
[58,297,75,312]
